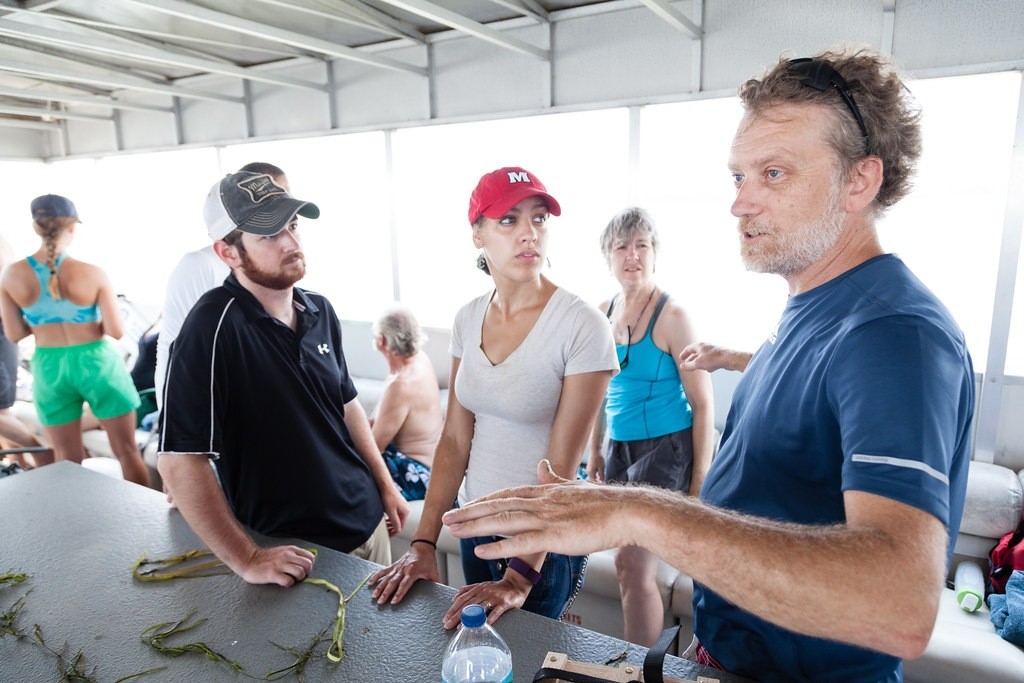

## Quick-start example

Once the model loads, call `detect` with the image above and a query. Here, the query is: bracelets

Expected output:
[506,556,541,585]
[409,539,437,550]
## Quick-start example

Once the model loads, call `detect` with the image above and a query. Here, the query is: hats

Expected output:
[468,167,561,224]
[30,194,82,223]
[203,171,320,241]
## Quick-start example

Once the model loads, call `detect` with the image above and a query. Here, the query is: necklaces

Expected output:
[614,285,655,339]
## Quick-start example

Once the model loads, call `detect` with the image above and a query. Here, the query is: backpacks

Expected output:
[984,516,1024,607]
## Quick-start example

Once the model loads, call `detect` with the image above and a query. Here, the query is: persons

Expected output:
[76,307,166,432]
[155,162,289,506]
[365,310,443,503]
[368,165,622,632]
[0,308,55,473]
[441,44,976,683]
[0,194,152,491]
[587,206,714,662]
[154,170,410,589]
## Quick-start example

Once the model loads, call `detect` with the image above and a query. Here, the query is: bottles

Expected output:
[441,604,513,683]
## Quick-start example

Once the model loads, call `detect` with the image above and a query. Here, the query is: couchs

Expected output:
[81,318,1024,683]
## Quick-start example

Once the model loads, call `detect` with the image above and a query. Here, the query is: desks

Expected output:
[0,457,745,683]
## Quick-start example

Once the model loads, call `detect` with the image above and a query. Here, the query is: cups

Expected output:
[955,561,985,612]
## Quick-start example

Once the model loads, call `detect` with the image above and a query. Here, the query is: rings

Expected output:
[482,600,492,608]
